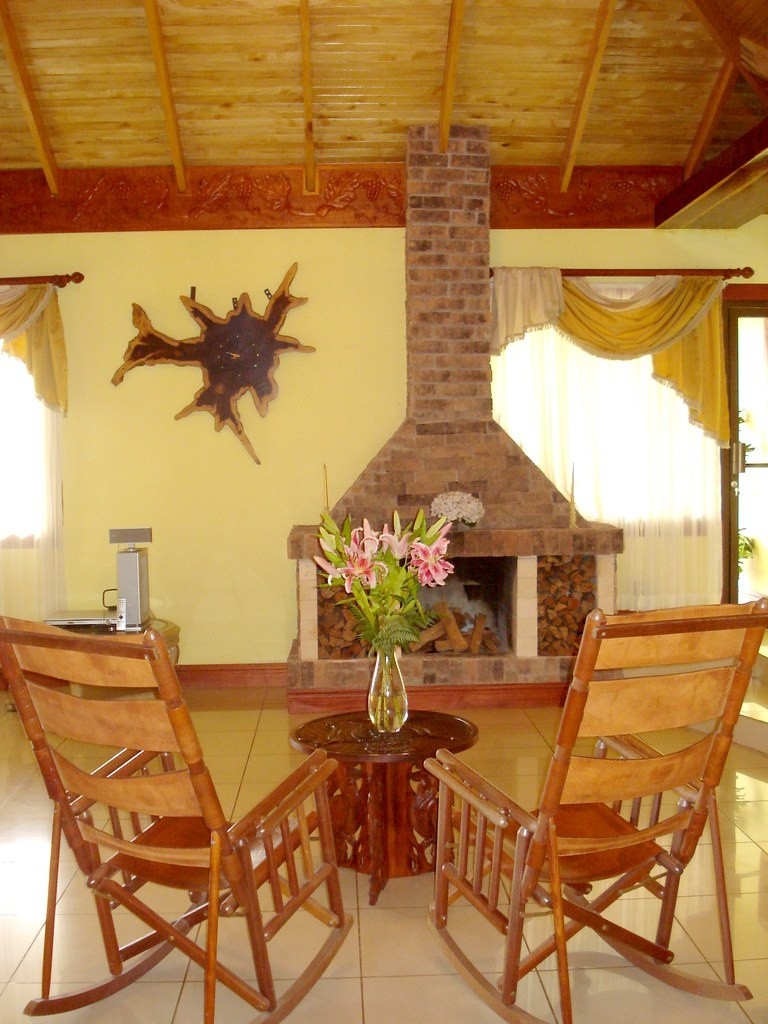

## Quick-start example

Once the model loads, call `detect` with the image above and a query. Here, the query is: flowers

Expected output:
[311,508,456,648]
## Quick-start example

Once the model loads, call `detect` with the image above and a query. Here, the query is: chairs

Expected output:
[0,616,354,1024]
[430,595,768,1024]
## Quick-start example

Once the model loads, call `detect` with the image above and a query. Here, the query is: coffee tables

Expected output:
[287,710,479,878]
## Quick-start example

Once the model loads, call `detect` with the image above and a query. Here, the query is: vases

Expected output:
[367,645,406,735]
